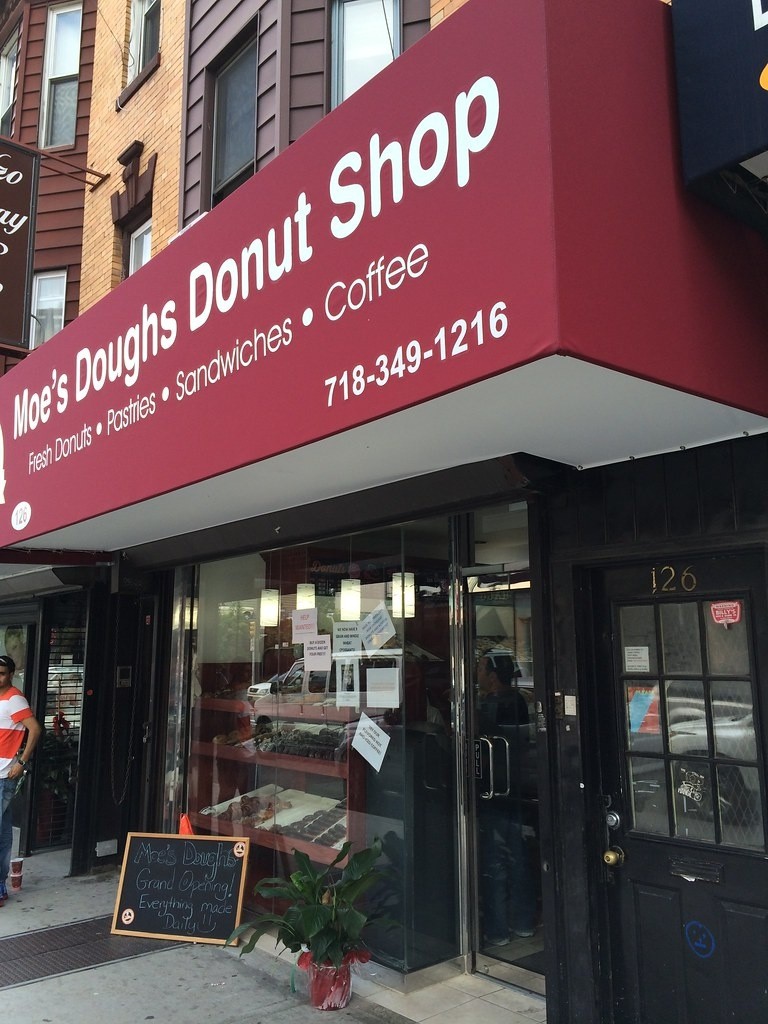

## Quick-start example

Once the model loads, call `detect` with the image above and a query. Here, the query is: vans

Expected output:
[252,648,403,726]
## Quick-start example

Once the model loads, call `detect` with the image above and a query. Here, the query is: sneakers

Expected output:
[507,924,537,937]
[483,933,510,945]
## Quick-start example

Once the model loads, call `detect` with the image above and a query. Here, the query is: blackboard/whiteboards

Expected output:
[109,831,250,947]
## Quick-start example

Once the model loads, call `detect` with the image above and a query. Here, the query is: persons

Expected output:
[474,646,539,945]
[193,650,256,855]
[4,624,27,694]
[0,654,40,907]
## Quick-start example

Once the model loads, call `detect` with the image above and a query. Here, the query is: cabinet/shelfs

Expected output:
[187,675,366,869]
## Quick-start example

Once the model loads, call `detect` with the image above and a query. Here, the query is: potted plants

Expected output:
[217,838,390,1010]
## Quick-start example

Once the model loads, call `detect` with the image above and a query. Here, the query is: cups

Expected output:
[11,875,22,890]
[10,858,24,874]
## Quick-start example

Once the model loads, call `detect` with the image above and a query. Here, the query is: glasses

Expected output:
[0,657,8,664]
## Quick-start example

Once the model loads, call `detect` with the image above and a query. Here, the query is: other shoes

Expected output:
[0,894,8,907]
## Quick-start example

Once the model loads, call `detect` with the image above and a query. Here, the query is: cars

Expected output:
[627,678,759,821]
[247,672,306,707]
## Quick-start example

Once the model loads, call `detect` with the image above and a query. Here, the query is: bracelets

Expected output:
[18,759,26,767]
